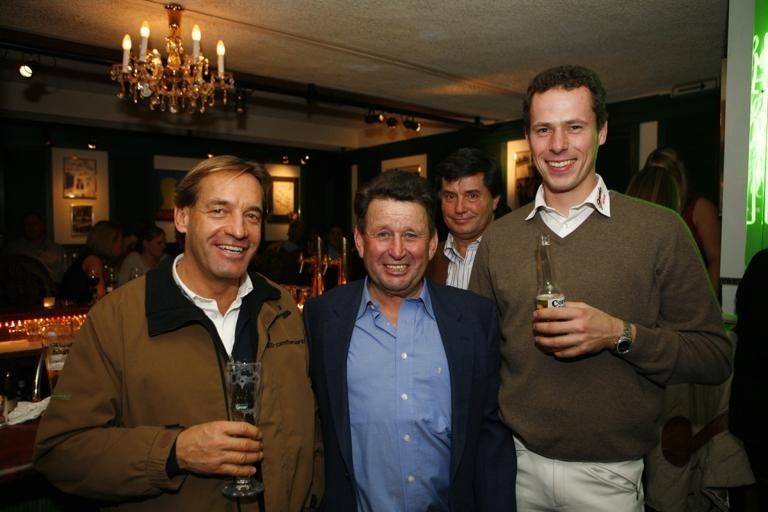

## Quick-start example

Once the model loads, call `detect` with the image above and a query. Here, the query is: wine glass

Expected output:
[222,359,267,500]
[88,268,100,306]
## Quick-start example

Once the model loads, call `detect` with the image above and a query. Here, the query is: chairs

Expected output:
[0,255,58,401]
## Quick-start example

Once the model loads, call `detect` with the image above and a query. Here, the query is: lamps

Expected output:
[368,107,423,134]
[110,3,237,117]
[18,60,41,80]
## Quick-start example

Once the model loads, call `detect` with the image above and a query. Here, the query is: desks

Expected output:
[0,396,56,507]
[0,306,92,359]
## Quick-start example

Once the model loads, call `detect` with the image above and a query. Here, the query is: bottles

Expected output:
[536,233,566,309]
[40,321,73,392]
[106,268,117,292]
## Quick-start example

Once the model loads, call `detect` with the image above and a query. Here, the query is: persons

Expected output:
[3,211,174,308]
[250,214,368,293]
[33,156,326,511]
[624,146,720,304]
[468,65,733,511]
[425,148,542,292]
[732,250,765,511]
[304,170,517,511]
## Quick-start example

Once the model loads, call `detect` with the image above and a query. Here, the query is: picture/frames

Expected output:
[379,153,429,181]
[507,138,537,209]
[269,175,300,224]
[154,168,190,222]
[68,203,95,237]
[61,157,99,199]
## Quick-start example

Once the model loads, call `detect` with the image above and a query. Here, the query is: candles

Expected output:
[297,231,348,267]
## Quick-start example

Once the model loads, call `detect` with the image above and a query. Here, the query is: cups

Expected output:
[131,267,144,279]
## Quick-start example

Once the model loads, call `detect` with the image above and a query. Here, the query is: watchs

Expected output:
[616,317,634,360]
